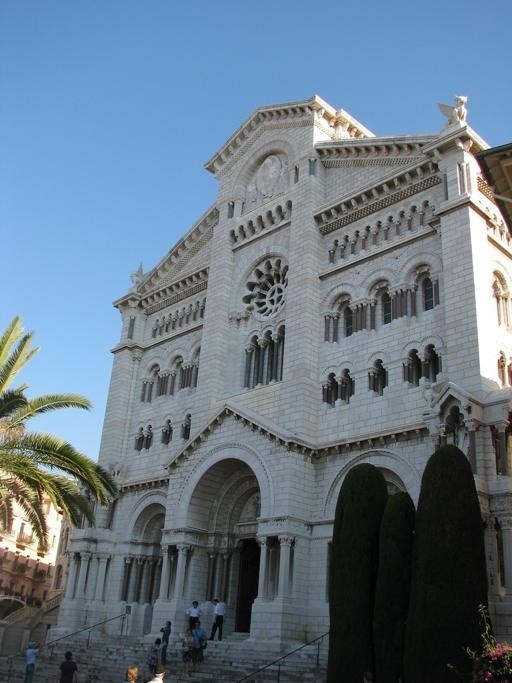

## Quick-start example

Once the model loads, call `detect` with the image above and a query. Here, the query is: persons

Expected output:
[145,663,166,682]
[186,600,203,635]
[59,650,79,682]
[122,664,139,682]
[23,640,40,682]
[181,628,194,677]
[146,637,161,673]
[207,598,226,641]
[192,619,208,665]
[362,670,374,682]
[160,621,171,664]
[393,671,404,682]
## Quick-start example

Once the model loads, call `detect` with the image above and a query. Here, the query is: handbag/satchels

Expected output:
[201,639,206,648]
